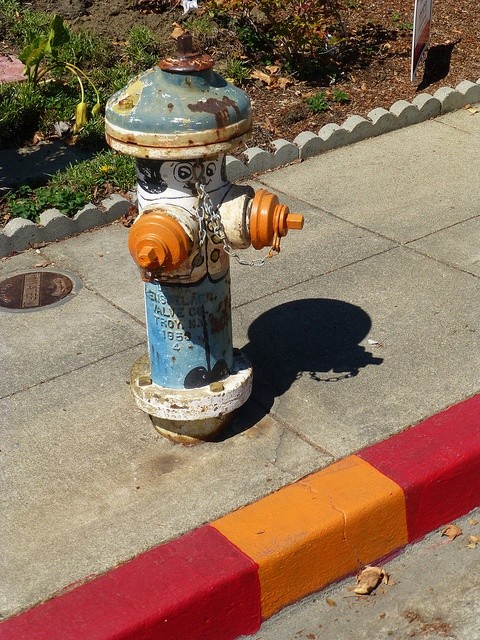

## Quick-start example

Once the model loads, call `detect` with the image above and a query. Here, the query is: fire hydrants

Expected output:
[104,33,304,446]
